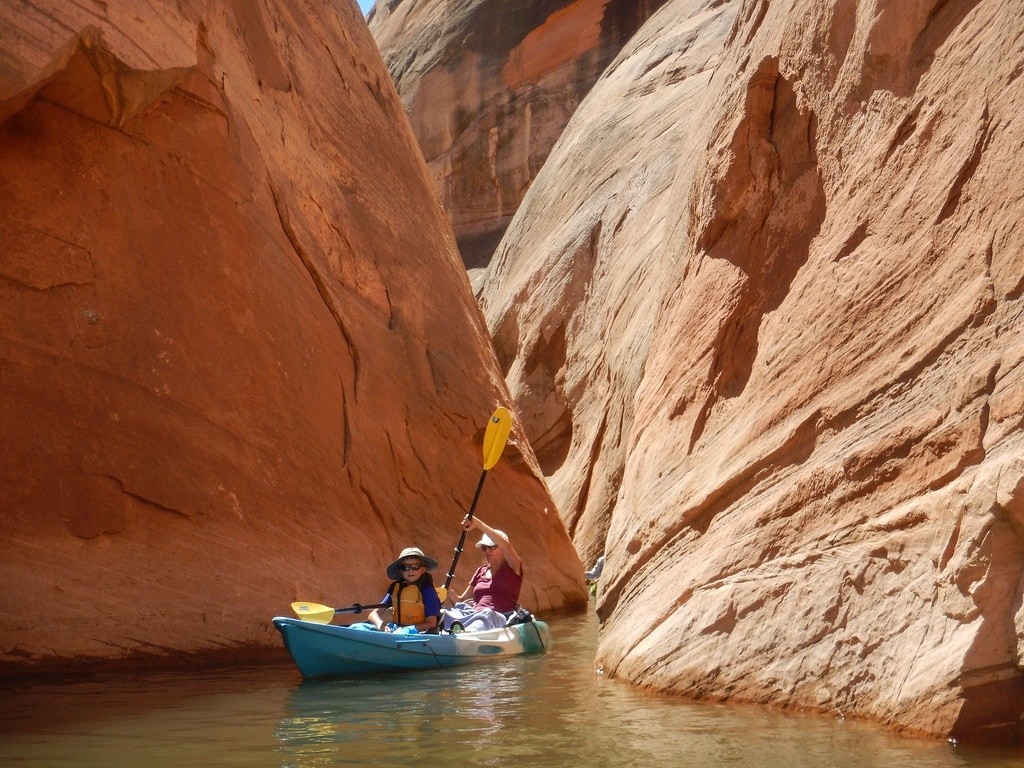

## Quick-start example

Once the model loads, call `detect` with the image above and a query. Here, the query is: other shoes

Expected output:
[449,620,465,635]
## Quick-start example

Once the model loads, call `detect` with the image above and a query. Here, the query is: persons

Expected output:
[440,513,523,635]
[347,547,441,636]
[585,555,605,580]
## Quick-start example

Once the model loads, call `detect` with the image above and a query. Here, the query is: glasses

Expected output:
[482,544,498,551]
[398,563,424,571]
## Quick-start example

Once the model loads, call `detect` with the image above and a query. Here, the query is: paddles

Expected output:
[435,407,513,600]
[289,584,449,626]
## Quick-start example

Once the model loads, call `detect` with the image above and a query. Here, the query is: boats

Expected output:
[271,617,551,682]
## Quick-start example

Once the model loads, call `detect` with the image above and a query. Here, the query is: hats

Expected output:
[475,529,508,549]
[387,547,438,580]
